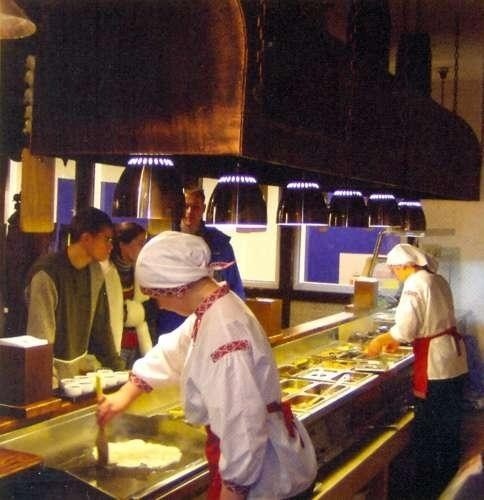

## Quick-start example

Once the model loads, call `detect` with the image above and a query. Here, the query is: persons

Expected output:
[367,243,468,499]
[95,230,317,500]
[110,221,145,370]
[154,186,244,347]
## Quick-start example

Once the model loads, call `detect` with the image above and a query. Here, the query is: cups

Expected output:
[57,367,130,402]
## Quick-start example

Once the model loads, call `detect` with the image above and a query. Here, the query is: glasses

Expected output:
[102,236,113,243]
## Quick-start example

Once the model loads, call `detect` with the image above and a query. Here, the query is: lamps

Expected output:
[108,153,187,227]
[396,196,430,239]
[199,159,268,233]
[327,180,369,229]
[273,166,330,233]
[364,185,405,235]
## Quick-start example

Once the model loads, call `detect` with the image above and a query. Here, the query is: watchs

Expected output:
[23,207,126,391]
